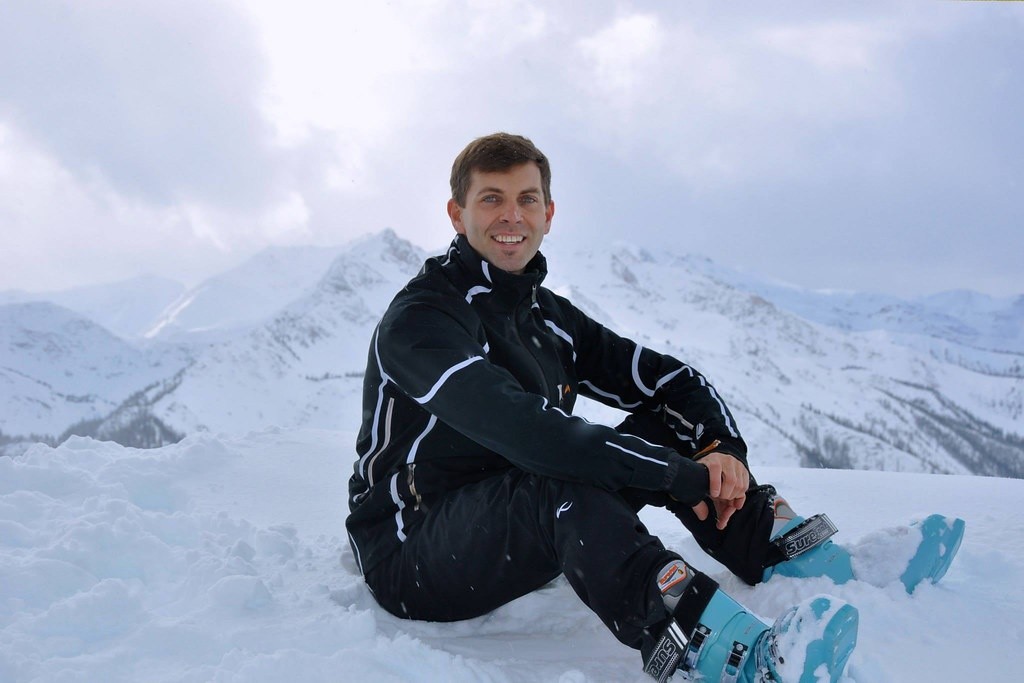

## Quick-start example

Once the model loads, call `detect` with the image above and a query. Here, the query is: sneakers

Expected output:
[643,551,859,683]
[758,483,966,599]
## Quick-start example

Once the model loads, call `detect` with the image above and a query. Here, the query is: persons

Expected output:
[346,133,965,682]
